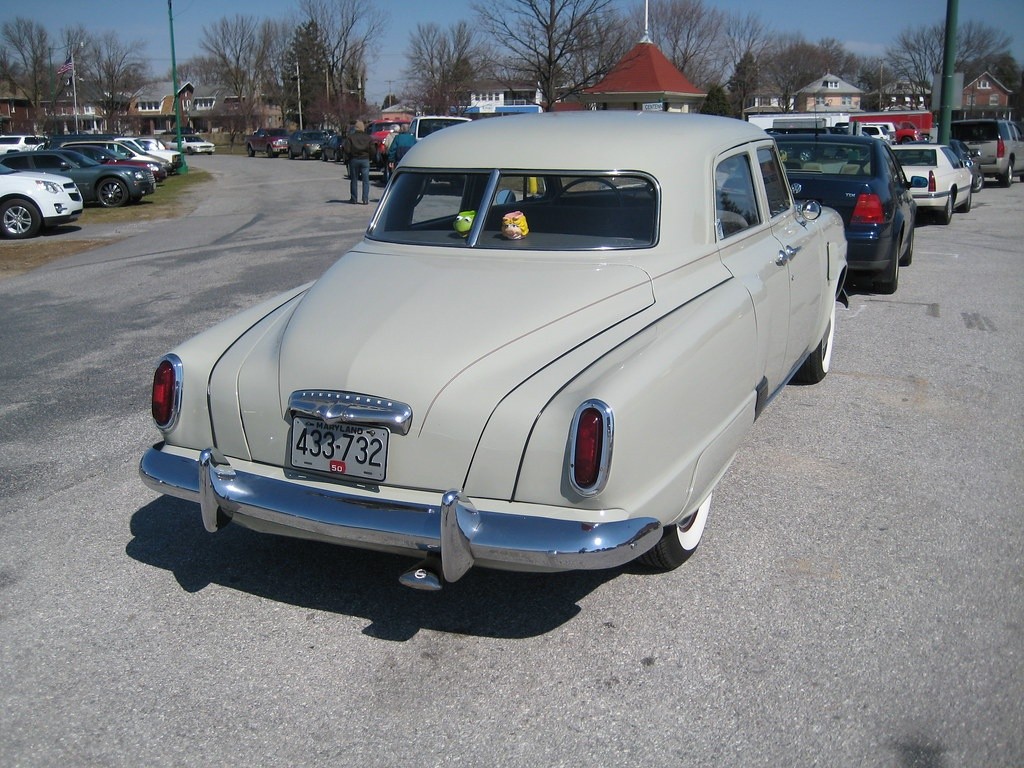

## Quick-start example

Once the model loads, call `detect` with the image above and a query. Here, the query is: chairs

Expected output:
[493,199,746,235]
[783,159,871,176]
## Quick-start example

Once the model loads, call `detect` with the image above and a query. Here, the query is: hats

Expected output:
[393,124,400,131]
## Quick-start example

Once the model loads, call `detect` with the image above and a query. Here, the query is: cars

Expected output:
[139,110,847,594]
[246,126,292,158]
[770,132,914,295]
[365,119,410,156]
[835,120,922,144]
[0,132,180,239]
[888,143,971,226]
[949,119,1024,188]
[949,139,985,193]
[287,130,337,160]
[391,115,469,152]
[165,135,215,155]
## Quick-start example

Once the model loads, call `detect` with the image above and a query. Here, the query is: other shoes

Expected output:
[350,199,357,203]
[363,200,368,205]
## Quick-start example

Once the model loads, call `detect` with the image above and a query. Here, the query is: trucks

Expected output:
[849,111,932,142]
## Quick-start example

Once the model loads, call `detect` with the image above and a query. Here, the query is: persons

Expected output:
[384,125,400,149]
[387,124,417,168]
[343,121,371,205]
